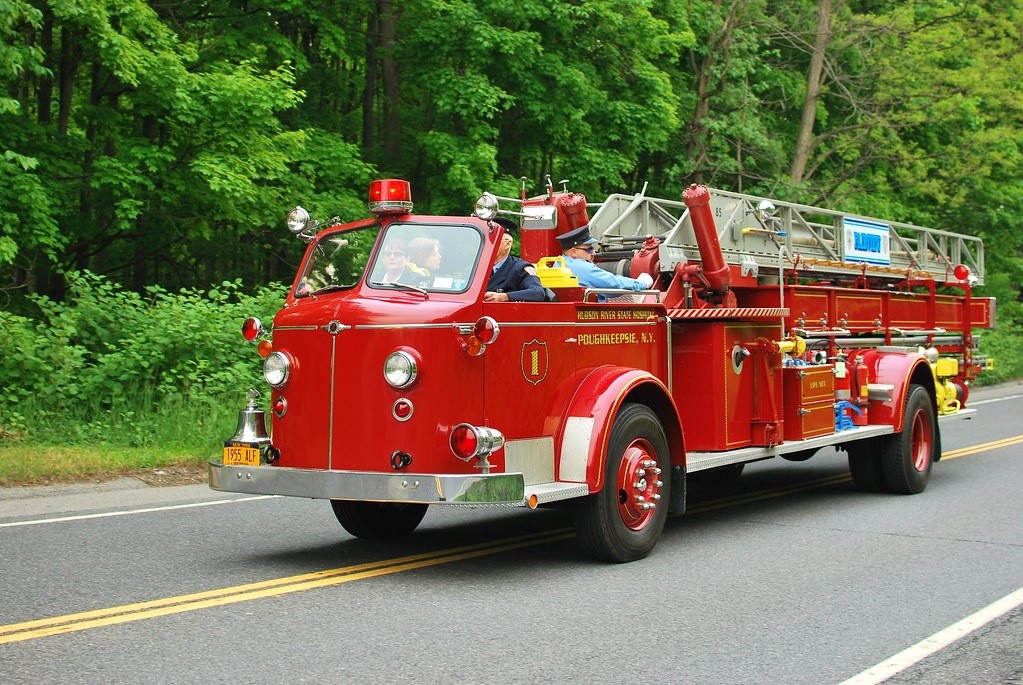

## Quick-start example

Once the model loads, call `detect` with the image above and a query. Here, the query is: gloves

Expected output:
[637,272,653,289]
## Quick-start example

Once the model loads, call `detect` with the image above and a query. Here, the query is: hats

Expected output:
[492,217,518,235]
[555,225,598,250]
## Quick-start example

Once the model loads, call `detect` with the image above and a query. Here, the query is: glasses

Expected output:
[382,251,406,256]
[576,247,594,253]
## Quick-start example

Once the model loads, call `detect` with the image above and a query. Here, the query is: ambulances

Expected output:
[205,180,995,567]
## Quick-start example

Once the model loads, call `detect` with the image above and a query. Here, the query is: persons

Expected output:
[378,236,441,287]
[553,225,653,303]
[461,217,546,302]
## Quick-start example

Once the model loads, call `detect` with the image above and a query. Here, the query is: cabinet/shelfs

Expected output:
[783,363,837,440]
